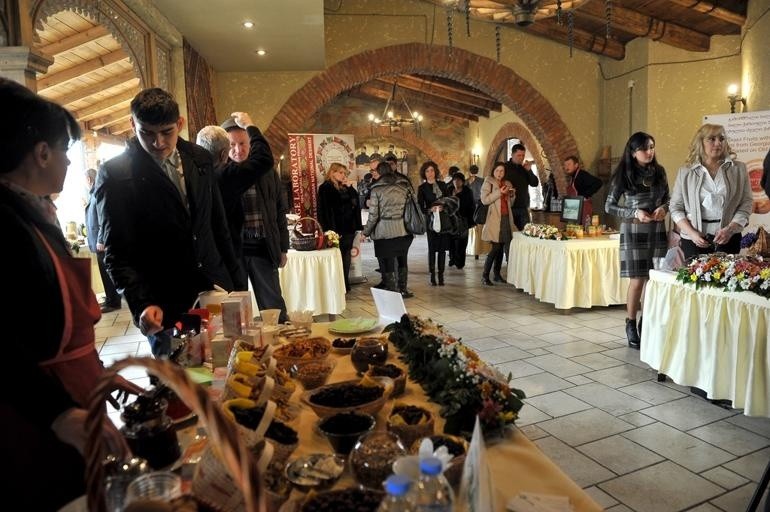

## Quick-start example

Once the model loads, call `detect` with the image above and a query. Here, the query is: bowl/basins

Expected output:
[286,453,348,487]
[290,359,337,391]
[315,412,377,457]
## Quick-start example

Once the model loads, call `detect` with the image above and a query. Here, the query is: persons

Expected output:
[80,167,123,315]
[670,122,753,271]
[192,111,273,299]
[604,132,670,349]
[95,86,245,362]
[0,75,147,512]
[563,155,603,225]
[221,116,290,326]
[315,144,545,299]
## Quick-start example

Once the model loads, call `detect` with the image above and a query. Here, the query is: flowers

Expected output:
[324,230,344,249]
[674,252,770,299]
[381,312,527,442]
[524,222,568,242]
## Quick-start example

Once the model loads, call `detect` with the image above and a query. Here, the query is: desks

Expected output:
[507,229,642,314]
[67,239,107,296]
[210,317,603,512]
[637,267,770,420]
[248,248,347,322]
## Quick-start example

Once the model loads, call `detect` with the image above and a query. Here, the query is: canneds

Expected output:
[575,226,583,238]
[592,214,599,226]
[587,225,596,237]
[596,225,602,237]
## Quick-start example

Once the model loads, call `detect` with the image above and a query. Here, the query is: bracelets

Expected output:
[726,224,735,235]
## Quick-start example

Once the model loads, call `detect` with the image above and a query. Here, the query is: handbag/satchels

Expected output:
[404,196,428,235]
[472,199,488,225]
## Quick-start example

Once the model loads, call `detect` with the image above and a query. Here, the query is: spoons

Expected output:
[420,437,454,474]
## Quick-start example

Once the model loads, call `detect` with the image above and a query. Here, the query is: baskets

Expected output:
[218,398,300,466]
[219,372,275,403]
[291,217,329,251]
[227,339,275,370]
[192,437,293,511]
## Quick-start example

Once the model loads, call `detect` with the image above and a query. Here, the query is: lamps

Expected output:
[368,77,424,141]
[442,0,614,63]
[472,136,485,165]
[727,82,747,117]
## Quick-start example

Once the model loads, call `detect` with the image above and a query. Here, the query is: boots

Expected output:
[399,272,414,298]
[428,253,445,286]
[382,271,396,292]
[492,256,506,283]
[480,255,494,286]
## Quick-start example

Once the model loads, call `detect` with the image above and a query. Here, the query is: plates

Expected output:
[328,318,379,334]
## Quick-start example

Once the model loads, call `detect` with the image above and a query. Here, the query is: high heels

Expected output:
[625,318,642,349]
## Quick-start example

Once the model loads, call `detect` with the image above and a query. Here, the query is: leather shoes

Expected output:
[100,304,121,313]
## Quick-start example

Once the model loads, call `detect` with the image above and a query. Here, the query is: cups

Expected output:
[285,319,313,331]
[261,309,282,326]
[126,470,186,505]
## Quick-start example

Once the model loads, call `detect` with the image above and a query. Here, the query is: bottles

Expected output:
[416,458,457,512]
[587,215,603,237]
[381,474,420,512]
[566,224,584,238]
